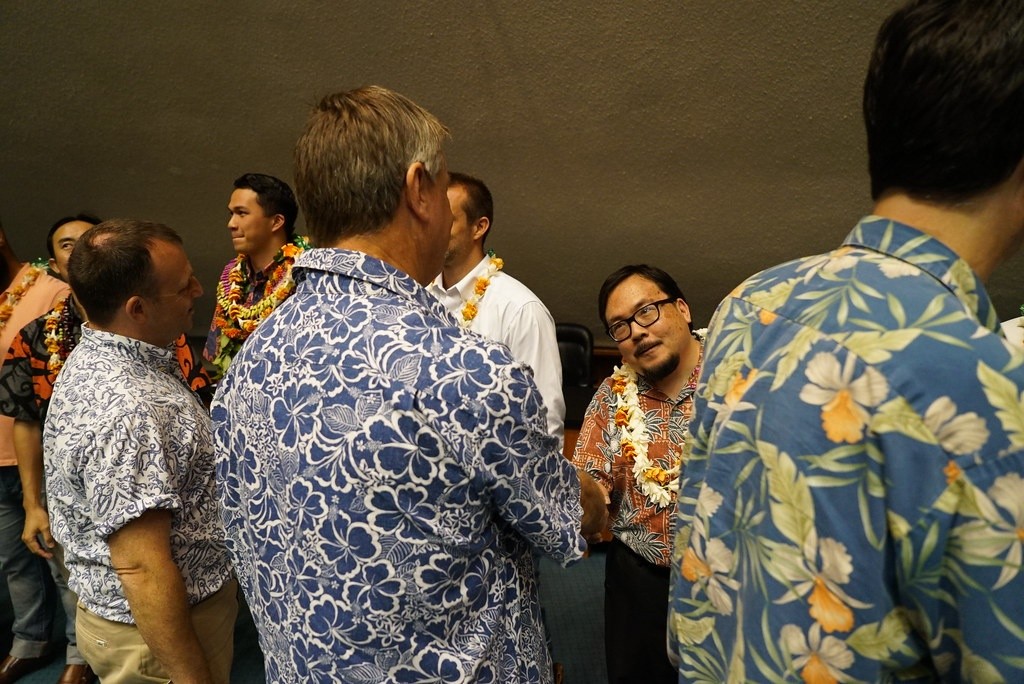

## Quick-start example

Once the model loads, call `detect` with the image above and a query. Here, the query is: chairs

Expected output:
[555,323,593,430]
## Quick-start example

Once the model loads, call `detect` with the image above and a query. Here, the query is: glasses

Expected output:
[606,298,674,343]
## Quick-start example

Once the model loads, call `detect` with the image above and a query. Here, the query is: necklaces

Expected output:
[0,261,47,329]
[212,235,310,375]
[460,248,504,328]
[42,295,74,377]
[611,328,705,505]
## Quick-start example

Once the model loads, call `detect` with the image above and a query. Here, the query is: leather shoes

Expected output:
[58,665,92,683]
[0,655,41,684]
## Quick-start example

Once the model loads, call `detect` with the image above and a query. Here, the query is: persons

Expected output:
[190,174,310,407]
[0,216,72,684]
[572,264,705,684]
[43,219,238,684]
[667,1,1024,684]
[209,84,608,684]
[423,172,566,454]
[0,216,195,683]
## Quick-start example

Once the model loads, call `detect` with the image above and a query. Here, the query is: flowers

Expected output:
[215,230,311,342]
[45,293,74,386]
[0,256,50,337]
[609,324,707,505]
[460,247,505,329]
